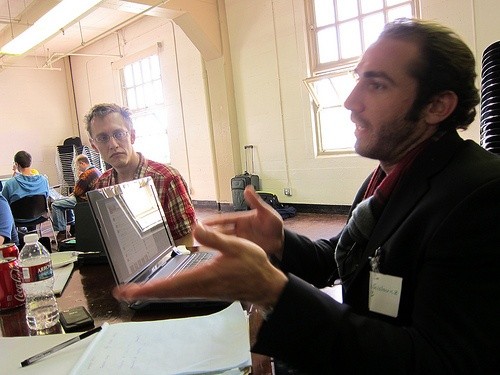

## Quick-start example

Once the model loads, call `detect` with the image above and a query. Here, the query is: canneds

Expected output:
[0,243,24,284]
[0,257,26,312]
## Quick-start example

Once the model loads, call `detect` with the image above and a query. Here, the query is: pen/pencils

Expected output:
[21,326,105,366]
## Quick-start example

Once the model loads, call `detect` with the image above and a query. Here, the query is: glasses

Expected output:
[89,129,129,143]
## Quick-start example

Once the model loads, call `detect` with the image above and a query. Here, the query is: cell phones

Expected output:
[58,306,94,333]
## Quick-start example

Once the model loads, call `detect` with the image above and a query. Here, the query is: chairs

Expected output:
[0,136,101,258]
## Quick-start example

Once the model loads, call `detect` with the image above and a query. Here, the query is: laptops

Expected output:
[0,179,10,192]
[75,176,236,305]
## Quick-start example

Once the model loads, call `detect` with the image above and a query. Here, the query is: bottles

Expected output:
[17,234,59,331]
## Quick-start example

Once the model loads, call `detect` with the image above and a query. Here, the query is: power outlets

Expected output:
[284,188,292,196]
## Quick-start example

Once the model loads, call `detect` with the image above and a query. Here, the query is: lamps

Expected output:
[0,0,106,56]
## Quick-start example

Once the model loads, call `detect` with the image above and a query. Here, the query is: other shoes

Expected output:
[51,231,71,243]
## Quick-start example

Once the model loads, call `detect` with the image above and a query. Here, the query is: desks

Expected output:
[0,247,277,375]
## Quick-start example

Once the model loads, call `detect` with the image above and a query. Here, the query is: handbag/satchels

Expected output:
[252,190,296,219]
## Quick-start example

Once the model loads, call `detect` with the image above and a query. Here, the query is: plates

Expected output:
[50,251,84,269]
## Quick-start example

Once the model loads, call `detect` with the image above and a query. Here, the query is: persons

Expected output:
[85,103,195,247]
[3,151,50,231]
[0,181,20,248]
[116,18,500,375]
[52,153,104,239]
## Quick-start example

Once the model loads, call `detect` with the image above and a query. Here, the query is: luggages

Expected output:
[230,145,259,212]
